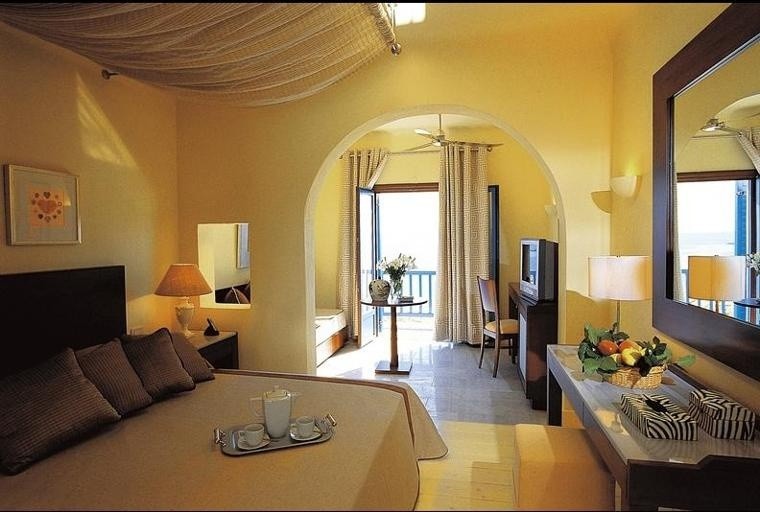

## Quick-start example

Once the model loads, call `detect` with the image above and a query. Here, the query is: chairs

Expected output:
[477,276,519,378]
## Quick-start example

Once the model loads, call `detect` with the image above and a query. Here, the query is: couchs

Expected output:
[315,307,348,367]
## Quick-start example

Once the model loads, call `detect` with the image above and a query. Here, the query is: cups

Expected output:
[239,424,264,446]
[296,415,314,436]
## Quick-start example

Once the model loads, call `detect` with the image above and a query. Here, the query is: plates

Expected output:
[238,433,271,450]
[290,426,321,441]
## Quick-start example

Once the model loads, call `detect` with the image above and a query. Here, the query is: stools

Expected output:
[511,423,615,511]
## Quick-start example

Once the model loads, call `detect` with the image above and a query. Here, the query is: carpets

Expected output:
[412,421,513,512]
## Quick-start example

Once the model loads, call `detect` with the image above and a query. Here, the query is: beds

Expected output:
[0,265,448,511]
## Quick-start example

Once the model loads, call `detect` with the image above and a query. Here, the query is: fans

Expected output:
[404,114,503,151]
[699,119,743,135]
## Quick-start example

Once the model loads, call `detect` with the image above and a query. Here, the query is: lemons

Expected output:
[610,348,641,368]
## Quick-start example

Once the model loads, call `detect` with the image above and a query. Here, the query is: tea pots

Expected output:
[249,385,303,437]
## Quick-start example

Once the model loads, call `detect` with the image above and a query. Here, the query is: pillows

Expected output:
[225,285,250,304]
[0,329,215,476]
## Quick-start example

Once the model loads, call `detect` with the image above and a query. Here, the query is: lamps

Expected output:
[688,255,745,312]
[610,176,636,198]
[154,264,213,340]
[589,257,650,333]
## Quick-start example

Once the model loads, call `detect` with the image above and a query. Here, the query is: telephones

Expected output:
[204,317,219,336]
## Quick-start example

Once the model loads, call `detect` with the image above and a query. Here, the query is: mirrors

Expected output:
[652,2,760,383]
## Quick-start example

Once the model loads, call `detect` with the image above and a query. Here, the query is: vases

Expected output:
[368,279,389,301]
[389,279,404,300]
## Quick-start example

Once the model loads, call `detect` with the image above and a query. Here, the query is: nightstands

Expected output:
[188,331,238,368]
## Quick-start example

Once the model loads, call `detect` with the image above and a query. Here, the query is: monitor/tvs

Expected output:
[519,238,558,306]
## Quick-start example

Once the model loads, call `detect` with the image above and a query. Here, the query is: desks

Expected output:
[548,345,760,512]
[360,296,428,374]
[508,282,557,411]
[732,299,760,308]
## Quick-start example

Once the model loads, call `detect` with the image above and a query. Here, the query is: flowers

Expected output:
[376,252,418,294]
[746,251,760,276]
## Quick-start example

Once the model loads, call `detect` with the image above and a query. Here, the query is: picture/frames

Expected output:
[238,222,248,266]
[3,164,81,245]
